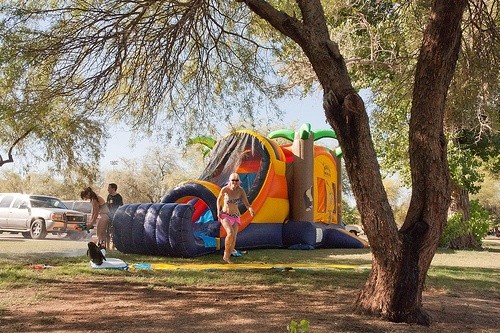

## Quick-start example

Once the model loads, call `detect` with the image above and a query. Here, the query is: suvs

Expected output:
[0,192,88,240]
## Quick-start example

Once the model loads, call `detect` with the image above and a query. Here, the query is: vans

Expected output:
[53,200,102,229]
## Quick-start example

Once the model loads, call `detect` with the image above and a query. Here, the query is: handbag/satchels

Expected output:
[87,242,106,265]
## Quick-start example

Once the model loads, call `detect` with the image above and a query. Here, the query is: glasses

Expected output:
[231,179,239,182]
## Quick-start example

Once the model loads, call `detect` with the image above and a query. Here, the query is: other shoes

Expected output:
[223,257,234,264]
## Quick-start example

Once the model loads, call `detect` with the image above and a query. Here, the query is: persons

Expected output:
[216,173,255,263]
[80,187,111,249]
[107,183,124,250]
